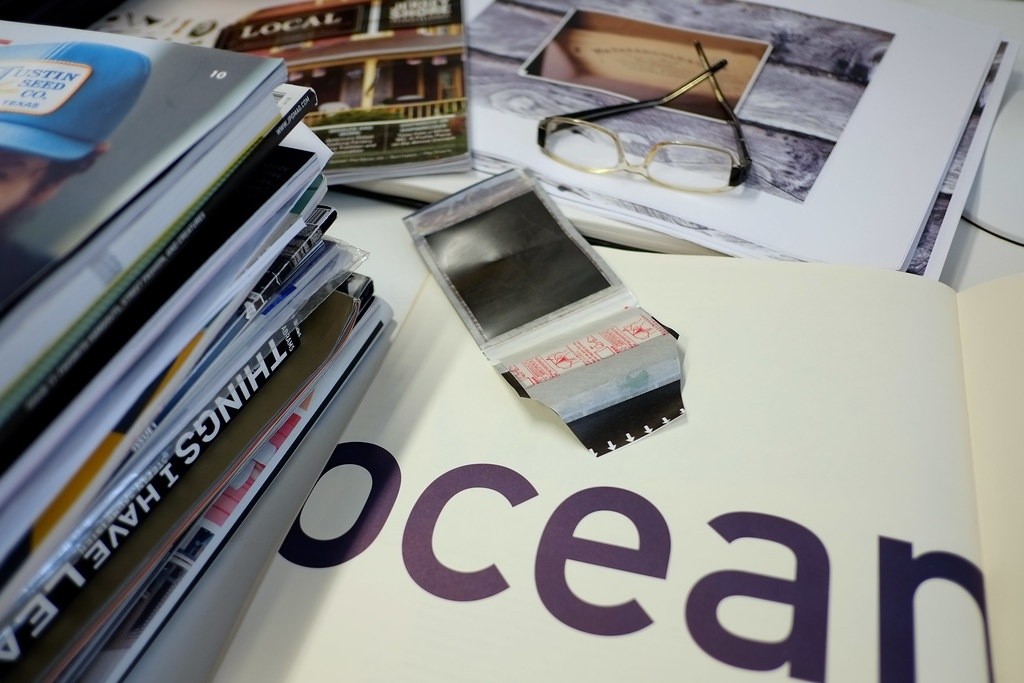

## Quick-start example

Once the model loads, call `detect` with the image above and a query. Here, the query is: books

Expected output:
[214,0,472,185]
[0,20,395,683]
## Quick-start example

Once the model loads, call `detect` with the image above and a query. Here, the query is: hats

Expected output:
[0,41,151,162]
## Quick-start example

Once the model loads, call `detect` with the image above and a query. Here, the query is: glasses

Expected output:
[537,40,753,195]
